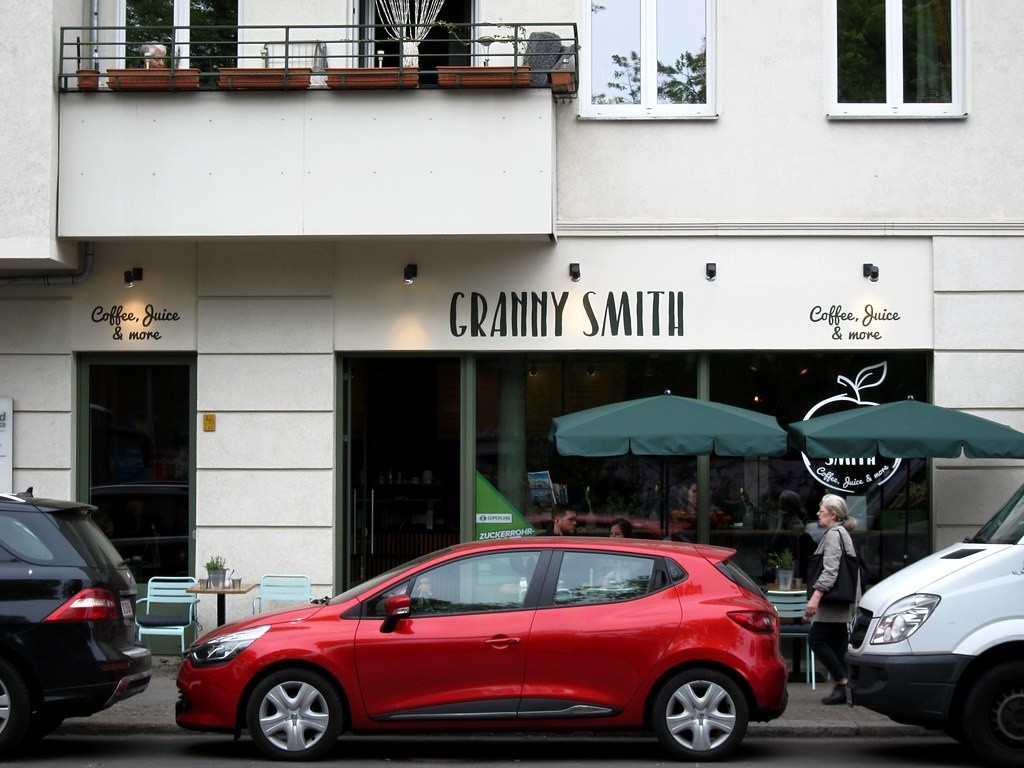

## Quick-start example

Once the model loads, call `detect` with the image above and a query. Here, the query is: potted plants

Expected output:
[203,556,227,589]
[768,550,796,589]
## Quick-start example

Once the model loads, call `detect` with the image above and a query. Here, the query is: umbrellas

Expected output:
[548,390,788,540]
[788,395,1024,568]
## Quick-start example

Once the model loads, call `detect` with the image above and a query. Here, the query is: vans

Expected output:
[843,484,1024,768]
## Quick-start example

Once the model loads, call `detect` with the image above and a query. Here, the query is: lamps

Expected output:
[863,263,879,282]
[403,263,419,284]
[569,264,580,282]
[124,268,145,288]
[707,263,716,281]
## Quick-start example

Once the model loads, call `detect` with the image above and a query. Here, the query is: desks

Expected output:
[186,583,259,628]
[761,581,828,682]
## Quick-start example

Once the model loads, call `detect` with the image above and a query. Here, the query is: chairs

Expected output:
[765,589,814,685]
[252,575,311,621]
[131,577,196,656]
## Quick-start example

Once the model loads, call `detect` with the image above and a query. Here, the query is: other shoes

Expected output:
[821,683,848,705]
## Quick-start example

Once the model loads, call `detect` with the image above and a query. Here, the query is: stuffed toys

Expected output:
[418,578,433,598]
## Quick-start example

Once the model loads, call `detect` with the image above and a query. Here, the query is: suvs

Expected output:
[0,484,153,765]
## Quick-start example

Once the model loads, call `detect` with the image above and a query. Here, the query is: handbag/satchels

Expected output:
[808,529,858,607]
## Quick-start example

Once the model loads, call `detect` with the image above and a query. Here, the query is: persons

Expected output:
[671,476,732,528]
[609,518,632,539]
[140,41,167,68]
[510,501,594,604]
[804,493,861,705]
[773,489,804,557]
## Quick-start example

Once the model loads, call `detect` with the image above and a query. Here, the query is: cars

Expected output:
[175,535,789,762]
[526,516,694,541]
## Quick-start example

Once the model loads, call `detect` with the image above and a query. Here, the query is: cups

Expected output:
[231,578,242,589]
[199,578,208,589]
[793,579,802,587]
